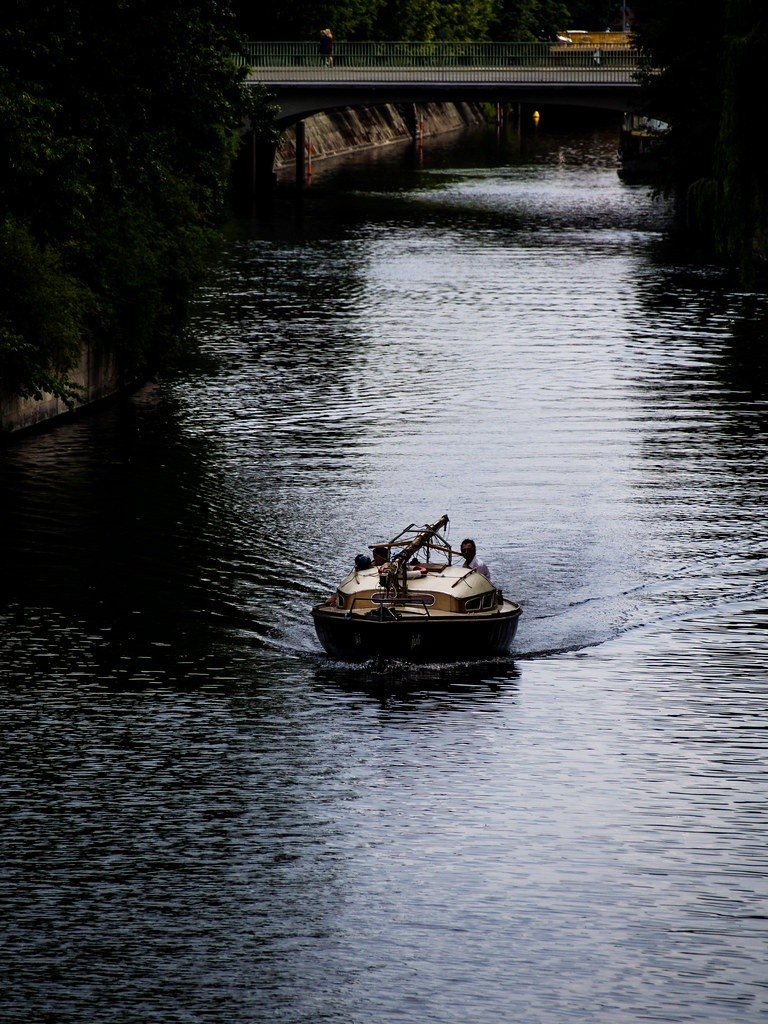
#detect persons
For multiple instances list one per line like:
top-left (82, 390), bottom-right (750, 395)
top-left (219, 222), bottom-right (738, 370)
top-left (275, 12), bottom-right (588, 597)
top-left (355, 554), bottom-right (372, 570)
top-left (461, 539), bottom-right (490, 580)
top-left (372, 547), bottom-right (391, 566)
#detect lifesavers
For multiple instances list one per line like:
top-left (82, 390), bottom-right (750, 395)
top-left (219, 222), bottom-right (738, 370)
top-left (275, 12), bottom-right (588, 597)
top-left (378, 562), bottom-right (427, 580)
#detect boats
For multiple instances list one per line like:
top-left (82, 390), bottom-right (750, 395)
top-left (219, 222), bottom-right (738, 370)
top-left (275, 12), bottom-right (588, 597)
top-left (306, 513), bottom-right (524, 662)
top-left (617, 110), bottom-right (669, 169)
